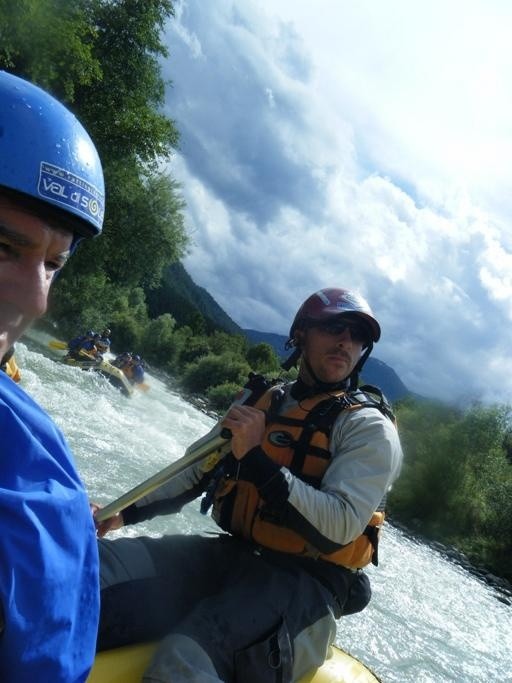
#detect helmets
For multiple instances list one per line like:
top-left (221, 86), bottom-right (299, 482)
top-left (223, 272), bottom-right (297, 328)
top-left (123, 352), bottom-right (144, 366)
top-left (0, 70), bottom-right (106, 256)
top-left (86, 329), bottom-right (111, 340)
top-left (290, 288), bottom-right (380, 351)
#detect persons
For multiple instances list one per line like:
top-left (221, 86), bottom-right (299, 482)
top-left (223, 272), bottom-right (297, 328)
top-left (1, 64), bottom-right (114, 683)
top-left (89, 288), bottom-right (402, 683)
top-left (61, 325), bottom-right (145, 388)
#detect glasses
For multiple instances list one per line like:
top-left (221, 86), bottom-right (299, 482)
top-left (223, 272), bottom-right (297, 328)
top-left (318, 317), bottom-right (370, 343)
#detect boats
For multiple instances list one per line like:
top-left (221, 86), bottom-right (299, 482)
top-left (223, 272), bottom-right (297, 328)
top-left (64, 352), bottom-right (133, 396)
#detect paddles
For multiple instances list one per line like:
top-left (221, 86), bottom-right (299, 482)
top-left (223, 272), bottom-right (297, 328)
top-left (137, 382), bottom-right (150, 392)
top-left (79, 348), bottom-right (97, 361)
top-left (49, 342), bottom-right (73, 350)
